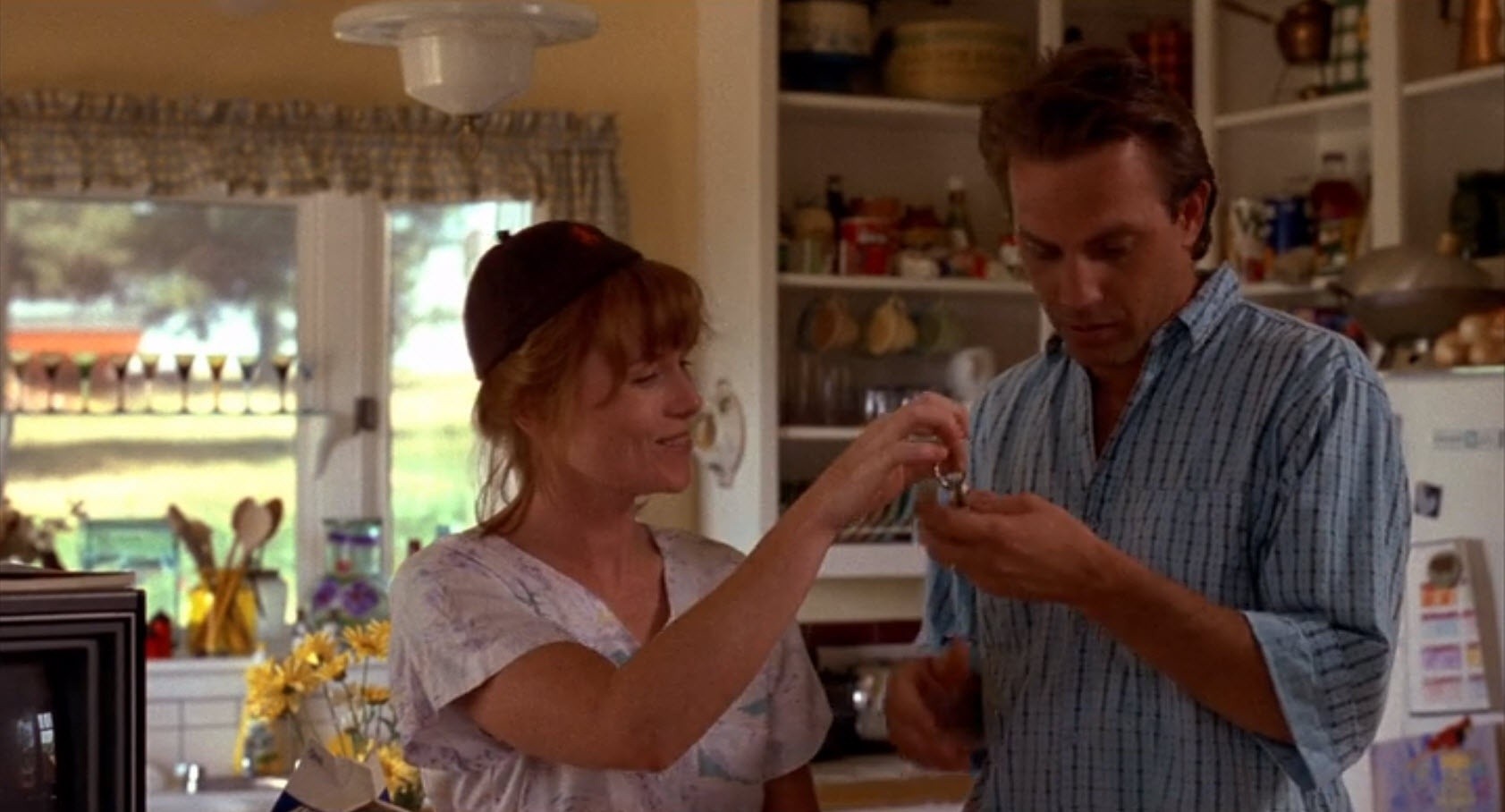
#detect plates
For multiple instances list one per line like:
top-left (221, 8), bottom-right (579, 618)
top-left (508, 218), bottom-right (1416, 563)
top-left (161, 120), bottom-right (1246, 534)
top-left (781, 463), bottom-right (922, 543)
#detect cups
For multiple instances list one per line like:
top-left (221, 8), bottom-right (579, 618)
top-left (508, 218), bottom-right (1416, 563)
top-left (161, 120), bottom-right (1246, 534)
top-left (783, 291), bottom-right (998, 427)
top-left (1229, 197), bottom-right (1268, 282)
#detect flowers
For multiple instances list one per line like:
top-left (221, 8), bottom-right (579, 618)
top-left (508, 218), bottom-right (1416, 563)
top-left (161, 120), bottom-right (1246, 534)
top-left (238, 615), bottom-right (425, 802)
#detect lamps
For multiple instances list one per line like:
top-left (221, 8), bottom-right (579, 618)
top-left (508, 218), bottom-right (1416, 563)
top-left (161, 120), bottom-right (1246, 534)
top-left (332, 1), bottom-right (600, 118)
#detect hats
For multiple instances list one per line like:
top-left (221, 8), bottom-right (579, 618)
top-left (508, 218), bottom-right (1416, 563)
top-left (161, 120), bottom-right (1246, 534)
top-left (463, 220), bottom-right (641, 377)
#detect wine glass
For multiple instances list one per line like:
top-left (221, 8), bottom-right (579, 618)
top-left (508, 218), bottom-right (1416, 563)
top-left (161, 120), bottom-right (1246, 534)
top-left (5, 351), bottom-right (35, 415)
top-left (107, 353), bottom-right (197, 415)
top-left (268, 355), bottom-right (294, 414)
top-left (237, 355), bottom-right (261, 416)
top-left (205, 354), bottom-right (227, 415)
top-left (39, 351), bottom-right (101, 415)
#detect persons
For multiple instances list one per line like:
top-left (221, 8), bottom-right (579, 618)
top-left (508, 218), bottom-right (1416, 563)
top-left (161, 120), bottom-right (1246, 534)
top-left (388, 219), bottom-right (968, 812)
top-left (886, 46), bottom-right (1413, 812)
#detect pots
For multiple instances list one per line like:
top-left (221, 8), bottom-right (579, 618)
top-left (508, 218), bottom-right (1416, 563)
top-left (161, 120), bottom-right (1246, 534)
top-left (1319, 231), bottom-right (1505, 346)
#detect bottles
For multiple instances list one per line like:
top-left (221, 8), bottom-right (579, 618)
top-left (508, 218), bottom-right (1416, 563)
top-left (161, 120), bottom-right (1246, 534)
top-left (781, 173), bottom-right (1030, 283)
top-left (119, 551), bottom-right (286, 659)
top-left (1263, 150), bottom-right (1364, 279)
top-left (304, 516), bottom-right (389, 632)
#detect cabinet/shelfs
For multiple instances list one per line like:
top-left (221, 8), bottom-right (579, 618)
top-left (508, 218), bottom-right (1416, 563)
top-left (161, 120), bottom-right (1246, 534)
top-left (694, 1), bottom-right (1505, 582)
